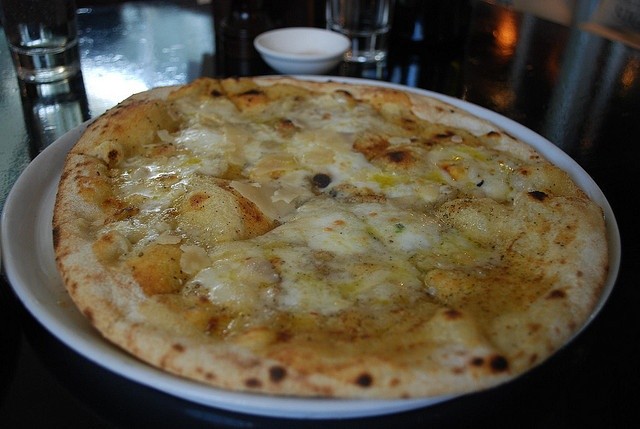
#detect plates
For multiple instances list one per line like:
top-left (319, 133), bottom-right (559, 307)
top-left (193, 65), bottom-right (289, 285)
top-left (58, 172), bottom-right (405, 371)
top-left (2, 74), bottom-right (622, 419)
top-left (253, 27), bottom-right (351, 74)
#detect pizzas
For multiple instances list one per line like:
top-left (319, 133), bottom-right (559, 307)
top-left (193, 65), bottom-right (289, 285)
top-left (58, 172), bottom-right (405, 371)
top-left (50, 73), bottom-right (608, 400)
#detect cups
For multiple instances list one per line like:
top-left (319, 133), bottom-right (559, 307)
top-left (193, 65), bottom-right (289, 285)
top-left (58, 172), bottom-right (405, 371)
top-left (0, 1), bottom-right (82, 85)
top-left (325, 0), bottom-right (389, 64)
